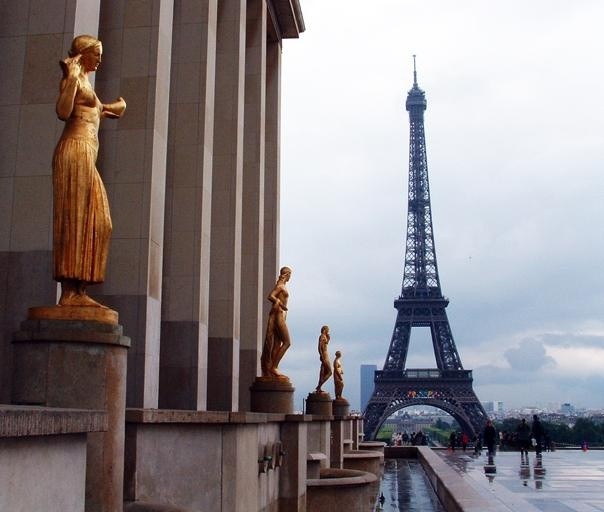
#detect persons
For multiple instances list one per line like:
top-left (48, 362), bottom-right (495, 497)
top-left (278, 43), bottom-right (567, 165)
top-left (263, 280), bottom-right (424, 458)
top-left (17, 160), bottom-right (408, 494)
top-left (52, 34), bottom-right (128, 307)
top-left (392, 429), bottom-right (428, 446)
top-left (446, 414), bottom-right (554, 456)
top-left (333, 350), bottom-right (345, 400)
top-left (580, 440), bottom-right (589, 452)
top-left (533, 453), bottom-right (546, 490)
top-left (484, 455), bottom-right (496, 484)
top-left (519, 449), bottom-right (531, 486)
top-left (315, 326), bottom-right (332, 392)
top-left (261, 266), bottom-right (291, 377)
